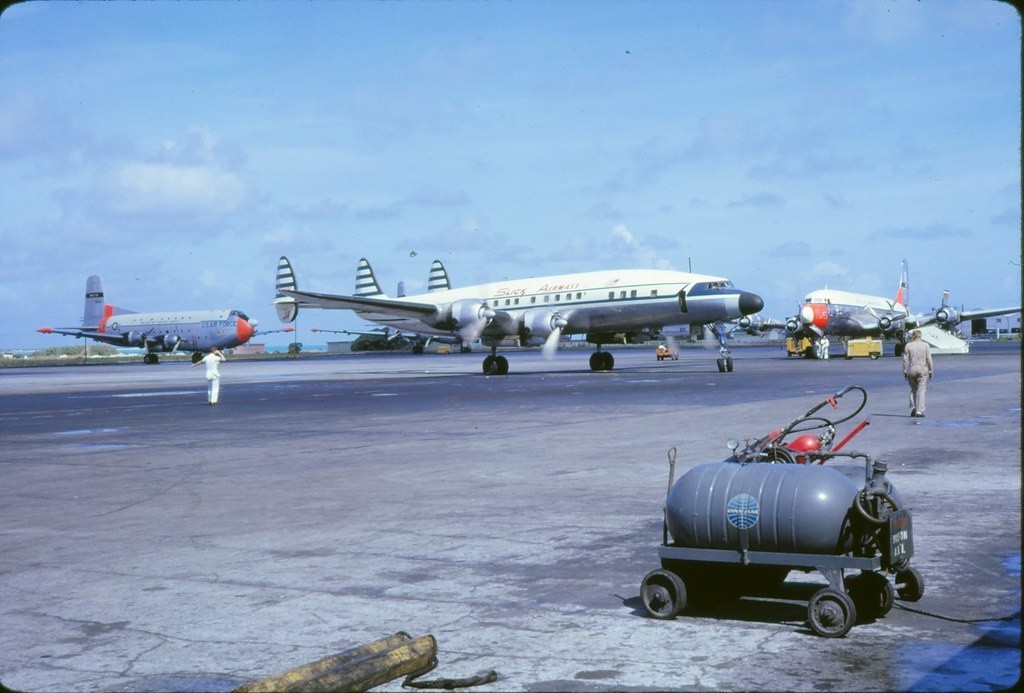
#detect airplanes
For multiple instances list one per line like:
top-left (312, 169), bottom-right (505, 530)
top-left (35, 275), bottom-right (258, 364)
top-left (728, 259), bottom-right (1021, 359)
top-left (272, 257), bottom-right (764, 374)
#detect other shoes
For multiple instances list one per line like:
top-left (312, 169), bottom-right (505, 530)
top-left (911, 408), bottom-right (925, 417)
top-left (209, 403), bottom-right (217, 406)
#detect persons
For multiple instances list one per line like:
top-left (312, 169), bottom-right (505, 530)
top-left (657, 343), bottom-right (666, 361)
top-left (820, 336), bottom-right (829, 360)
top-left (903, 330), bottom-right (934, 417)
top-left (812, 339), bottom-right (822, 358)
top-left (192, 347), bottom-right (226, 405)
